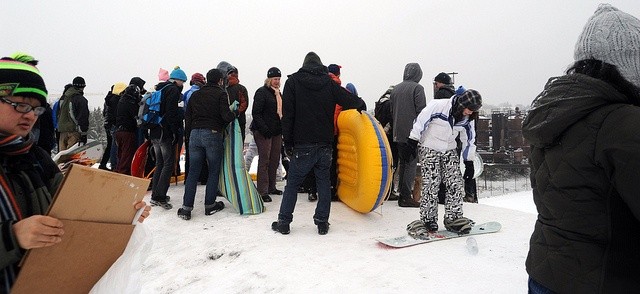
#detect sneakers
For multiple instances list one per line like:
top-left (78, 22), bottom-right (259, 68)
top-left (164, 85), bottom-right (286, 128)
top-left (178, 208), bottom-right (191, 220)
top-left (261, 193), bottom-right (272, 202)
top-left (314, 216), bottom-right (329, 234)
top-left (150, 196), bottom-right (170, 207)
top-left (444, 219), bottom-right (471, 233)
top-left (398, 197), bottom-right (419, 207)
top-left (308, 188), bottom-right (317, 200)
top-left (269, 189), bottom-right (283, 195)
top-left (424, 218), bottom-right (438, 231)
top-left (205, 200), bottom-right (225, 215)
top-left (272, 220), bottom-right (290, 234)
top-left (150, 196), bottom-right (172, 209)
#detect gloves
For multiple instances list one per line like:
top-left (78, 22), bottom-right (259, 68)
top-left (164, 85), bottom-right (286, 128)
top-left (78, 134), bottom-right (87, 147)
top-left (284, 146), bottom-right (294, 160)
top-left (463, 161), bottom-right (474, 183)
top-left (402, 138), bottom-right (418, 163)
top-left (356, 101), bottom-right (367, 114)
top-left (263, 130), bottom-right (272, 140)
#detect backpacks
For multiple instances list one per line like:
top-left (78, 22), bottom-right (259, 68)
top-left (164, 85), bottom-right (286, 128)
top-left (136, 83), bottom-right (181, 130)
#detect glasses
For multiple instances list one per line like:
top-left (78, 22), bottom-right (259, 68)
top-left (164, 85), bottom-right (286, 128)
top-left (0, 97), bottom-right (47, 116)
top-left (435, 83), bottom-right (437, 86)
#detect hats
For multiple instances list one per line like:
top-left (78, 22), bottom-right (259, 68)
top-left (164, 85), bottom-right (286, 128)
top-left (159, 68), bottom-right (169, 80)
top-left (0, 52), bottom-right (48, 107)
top-left (63, 84), bottom-right (72, 94)
top-left (434, 73), bottom-right (452, 84)
top-left (192, 73), bottom-right (204, 84)
top-left (268, 67), bottom-right (281, 78)
top-left (577, 3), bottom-right (640, 86)
top-left (458, 90), bottom-right (482, 110)
top-left (328, 64), bottom-right (340, 76)
top-left (169, 64), bottom-right (187, 82)
top-left (73, 77), bottom-right (86, 87)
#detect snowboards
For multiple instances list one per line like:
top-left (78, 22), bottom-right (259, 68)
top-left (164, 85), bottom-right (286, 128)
top-left (375, 221), bottom-right (501, 247)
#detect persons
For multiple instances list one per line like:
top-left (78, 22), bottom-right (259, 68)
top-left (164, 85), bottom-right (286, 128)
top-left (53, 84), bottom-right (73, 154)
top-left (97, 75), bottom-right (145, 176)
top-left (403, 89), bottom-right (482, 233)
top-left (271, 52), bottom-right (367, 235)
top-left (140, 67), bottom-right (186, 210)
top-left (309, 61), bottom-right (342, 203)
top-left (251, 67), bottom-right (283, 204)
top-left (520, 2), bottom-right (640, 294)
top-left (183, 72), bottom-right (206, 187)
top-left (376, 85), bottom-right (401, 201)
top-left (434, 73), bottom-right (478, 204)
top-left (392, 63), bottom-right (427, 207)
top-left (177, 69), bottom-right (226, 218)
top-left (58, 77), bottom-right (89, 153)
top-left (0, 51), bottom-right (151, 294)
top-left (218, 61), bottom-right (248, 152)
